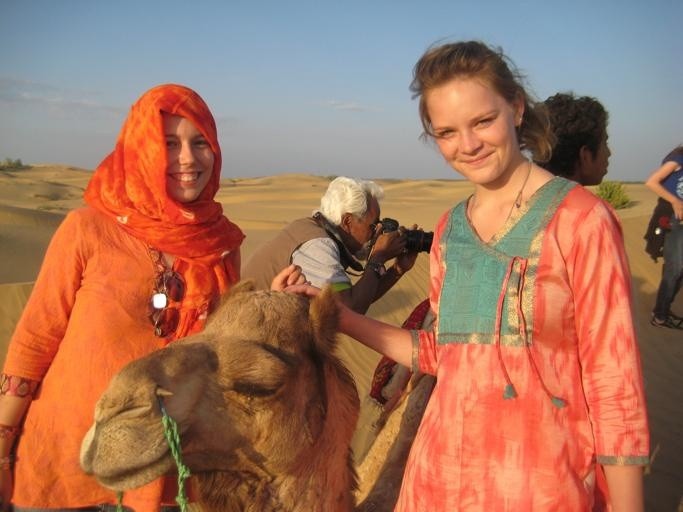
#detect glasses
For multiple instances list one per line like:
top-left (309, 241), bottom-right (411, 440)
top-left (148, 272), bottom-right (186, 337)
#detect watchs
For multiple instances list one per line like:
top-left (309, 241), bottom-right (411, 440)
top-left (363, 258), bottom-right (388, 279)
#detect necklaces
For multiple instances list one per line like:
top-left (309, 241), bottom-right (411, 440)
top-left (469, 161), bottom-right (532, 246)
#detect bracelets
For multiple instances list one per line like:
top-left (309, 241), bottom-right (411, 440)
top-left (1, 424), bottom-right (22, 440)
top-left (0, 455), bottom-right (13, 468)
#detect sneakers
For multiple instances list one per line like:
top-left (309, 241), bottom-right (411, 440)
top-left (652, 306), bottom-right (683, 329)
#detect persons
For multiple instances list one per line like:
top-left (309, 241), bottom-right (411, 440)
top-left (1, 81), bottom-right (314, 512)
top-left (279, 38), bottom-right (656, 512)
top-left (642, 138), bottom-right (683, 333)
top-left (239, 175), bottom-right (426, 316)
top-left (525, 88), bottom-right (614, 187)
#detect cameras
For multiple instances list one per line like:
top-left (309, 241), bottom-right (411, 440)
top-left (371, 217), bottom-right (434, 253)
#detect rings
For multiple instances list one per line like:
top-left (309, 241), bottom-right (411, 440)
top-left (400, 247), bottom-right (408, 257)
top-left (396, 228), bottom-right (404, 238)
